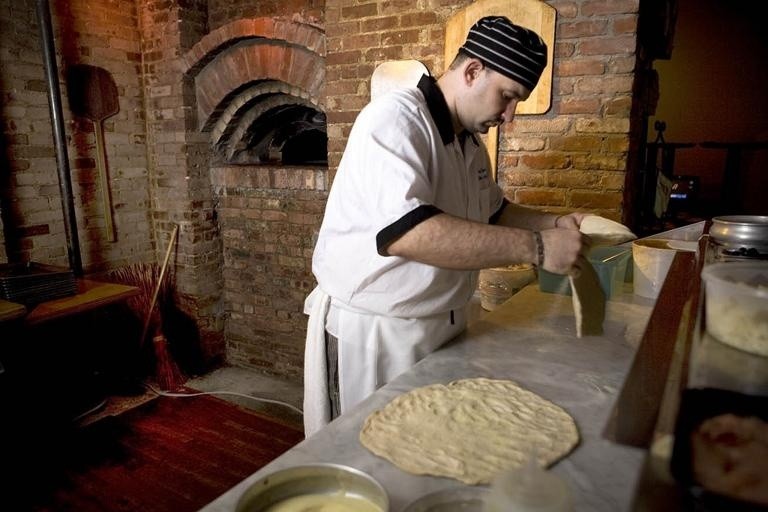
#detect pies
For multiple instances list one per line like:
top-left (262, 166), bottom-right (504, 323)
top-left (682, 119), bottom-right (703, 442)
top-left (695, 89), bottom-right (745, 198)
top-left (691, 413), bottom-right (768, 505)
top-left (567, 215), bottom-right (638, 339)
top-left (360, 377), bottom-right (579, 487)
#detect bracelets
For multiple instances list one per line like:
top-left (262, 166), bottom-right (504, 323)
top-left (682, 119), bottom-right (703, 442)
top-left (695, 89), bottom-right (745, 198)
top-left (534, 232), bottom-right (544, 269)
top-left (554, 211), bottom-right (576, 228)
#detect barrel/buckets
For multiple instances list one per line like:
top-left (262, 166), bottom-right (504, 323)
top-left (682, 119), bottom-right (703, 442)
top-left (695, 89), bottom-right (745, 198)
top-left (478, 267), bottom-right (535, 311)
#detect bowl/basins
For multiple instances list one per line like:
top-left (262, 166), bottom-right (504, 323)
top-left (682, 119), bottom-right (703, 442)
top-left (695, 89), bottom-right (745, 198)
top-left (232, 462), bottom-right (391, 512)
top-left (703, 213), bottom-right (768, 253)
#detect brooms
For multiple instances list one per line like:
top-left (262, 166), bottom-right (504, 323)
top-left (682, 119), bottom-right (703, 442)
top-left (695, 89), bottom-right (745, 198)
top-left (104, 264), bottom-right (189, 393)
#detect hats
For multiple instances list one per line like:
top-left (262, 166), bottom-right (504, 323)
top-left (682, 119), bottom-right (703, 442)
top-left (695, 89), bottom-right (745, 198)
top-left (457, 13), bottom-right (550, 92)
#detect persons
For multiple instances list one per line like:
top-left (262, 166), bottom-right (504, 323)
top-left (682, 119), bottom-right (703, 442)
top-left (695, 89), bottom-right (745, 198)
top-left (302, 16), bottom-right (594, 440)
